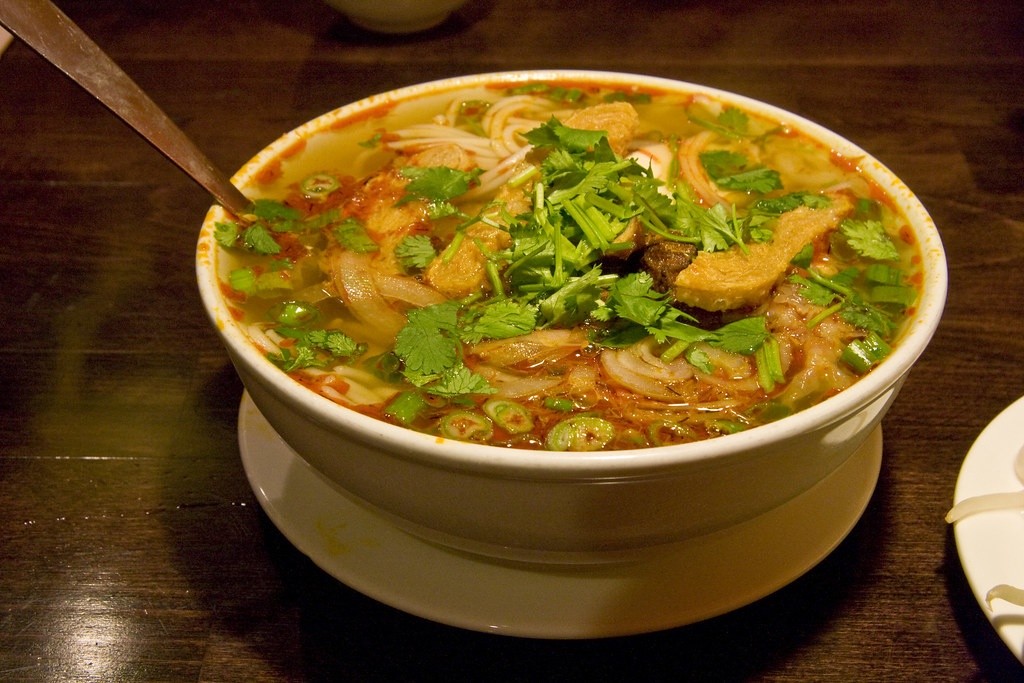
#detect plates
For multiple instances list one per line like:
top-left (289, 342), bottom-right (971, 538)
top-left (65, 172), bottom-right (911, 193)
top-left (952, 396), bottom-right (1024, 663)
top-left (240, 389), bottom-right (884, 638)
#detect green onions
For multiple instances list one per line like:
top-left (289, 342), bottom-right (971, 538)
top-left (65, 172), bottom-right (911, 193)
top-left (227, 171), bottom-right (916, 451)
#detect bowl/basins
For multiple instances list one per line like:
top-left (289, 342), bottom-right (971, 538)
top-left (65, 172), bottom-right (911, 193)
top-left (195, 70), bottom-right (949, 551)
top-left (329, 0), bottom-right (464, 34)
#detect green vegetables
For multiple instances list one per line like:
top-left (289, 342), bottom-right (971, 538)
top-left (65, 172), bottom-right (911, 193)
top-left (215, 86), bottom-right (900, 399)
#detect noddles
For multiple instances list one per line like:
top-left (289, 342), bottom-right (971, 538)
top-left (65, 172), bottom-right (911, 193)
top-left (214, 81), bottom-right (924, 451)
